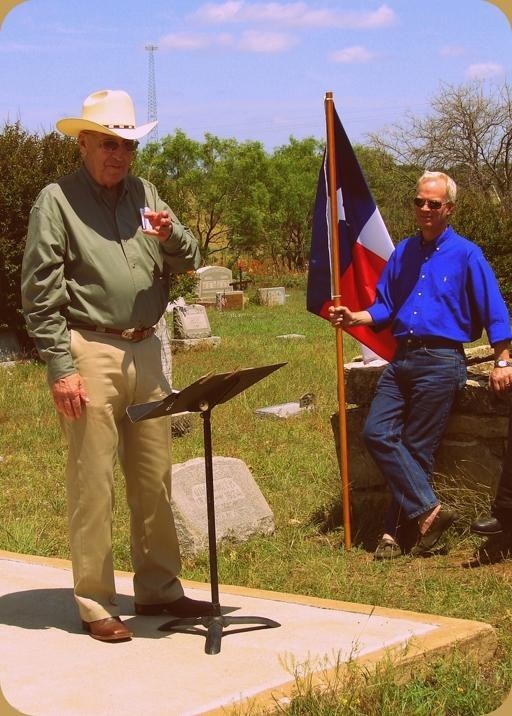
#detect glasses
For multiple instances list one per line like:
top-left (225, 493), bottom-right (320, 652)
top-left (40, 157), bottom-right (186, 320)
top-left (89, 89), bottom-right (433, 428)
top-left (410, 197), bottom-right (451, 209)
top-left (82, 131), bottom-right (141, 151)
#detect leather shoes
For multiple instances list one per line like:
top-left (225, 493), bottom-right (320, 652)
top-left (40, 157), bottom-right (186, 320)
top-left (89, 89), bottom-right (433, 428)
top-left (407, 502), bottom-right (458, 557)
top-left (135, 595), bottom-right (216, 616)
top-left (470, 515), bottom-right (509, 533)
top-left (374, 536), bottom-right (404, 561)
top-left (82, 614), bottom-right (134, 640)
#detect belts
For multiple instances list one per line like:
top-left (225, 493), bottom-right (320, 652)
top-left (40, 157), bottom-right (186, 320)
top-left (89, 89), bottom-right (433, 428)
top-left (398, 336), bottom-right (457, 349)
top-left (69, 314), bottom-right (165, 343)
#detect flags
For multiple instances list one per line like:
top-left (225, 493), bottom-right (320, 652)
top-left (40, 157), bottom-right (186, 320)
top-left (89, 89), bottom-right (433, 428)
top-left (307, 101), bottom-right (402, 365)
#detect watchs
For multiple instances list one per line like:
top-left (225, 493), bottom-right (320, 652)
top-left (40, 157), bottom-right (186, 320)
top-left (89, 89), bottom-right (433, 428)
top-left (493, 359), bottom-right (511, 368)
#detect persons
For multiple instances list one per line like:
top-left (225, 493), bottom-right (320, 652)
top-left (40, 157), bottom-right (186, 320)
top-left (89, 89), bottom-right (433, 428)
top-left (23, 91), bottom-right (219, 644)
top-left (471, 427), bottom-right (512, 537)
top-left (328, 170), bottom-right (512, 559)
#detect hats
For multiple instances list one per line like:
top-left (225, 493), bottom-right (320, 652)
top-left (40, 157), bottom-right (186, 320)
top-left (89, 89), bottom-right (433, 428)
top-left (57, 91), bottom-right (158, 138)
